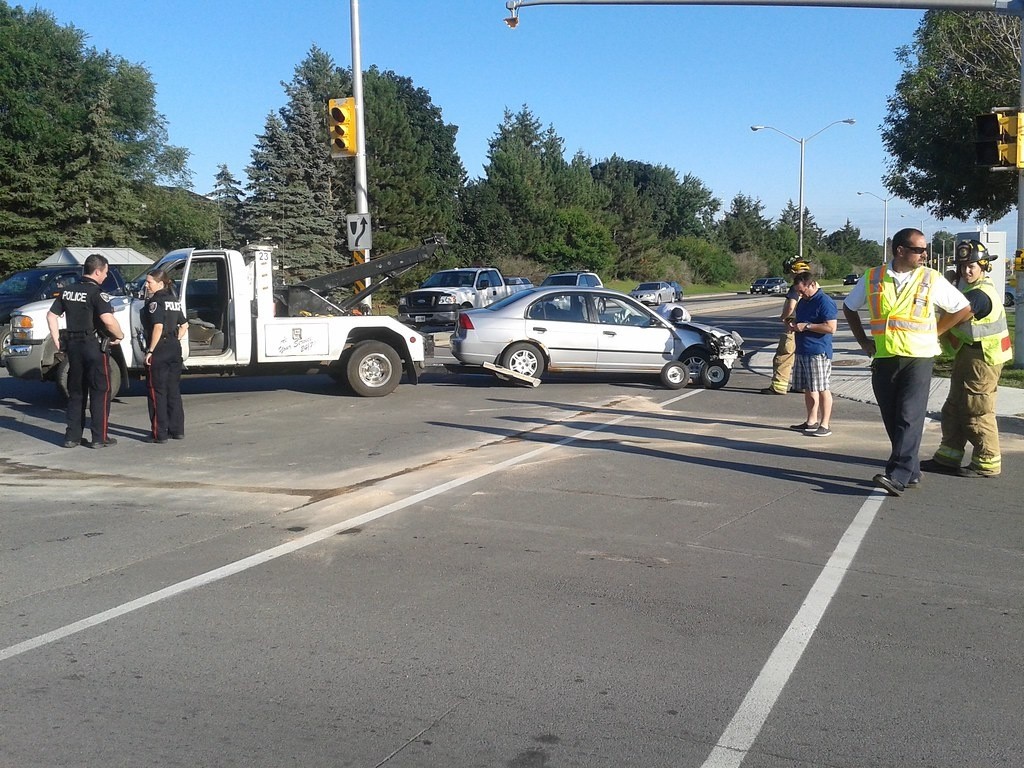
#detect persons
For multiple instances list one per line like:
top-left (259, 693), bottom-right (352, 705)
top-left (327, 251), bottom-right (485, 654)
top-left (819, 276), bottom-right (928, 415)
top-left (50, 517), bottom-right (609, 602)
top-left (46, 253), bottom-right (124, 449)
top-left (920, 239), bottom-right (1013, 478)
top-left (759, 254), bottom-right (837, 437)
top-left (656, 303), bottom-right (691, 323)
top-left (843, 228), bottom-right (970, 497)
top-left (140, 269), bottom-right (189, 444)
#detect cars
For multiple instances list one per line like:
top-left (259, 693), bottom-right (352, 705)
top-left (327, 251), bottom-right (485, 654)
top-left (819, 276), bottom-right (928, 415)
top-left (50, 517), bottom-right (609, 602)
top-left (749, 277), bottom-right (788, 295)
top-left (1003, 279), bottom-right (1016, 308)
top-left (842, 273), bottom-right (860, 286)
top-left (537, 269), bottom-right (607, 316)
top-left (626, 281), bottom-right (676, 306)
top-left (665, 281), bottom-right (684, 302)
top-left (449, 282), bottom-right (746, 391)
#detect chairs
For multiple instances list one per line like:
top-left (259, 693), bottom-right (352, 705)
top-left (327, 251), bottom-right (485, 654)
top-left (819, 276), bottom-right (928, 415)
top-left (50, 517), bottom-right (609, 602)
top-left (572, 301), bottom-right (586, 322)
top-left (189, 290), bottom-right (227, 356)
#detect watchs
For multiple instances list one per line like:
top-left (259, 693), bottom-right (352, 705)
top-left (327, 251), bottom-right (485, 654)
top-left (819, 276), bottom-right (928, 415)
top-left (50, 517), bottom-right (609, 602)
top-left (807, 323), bottom-right (811, 330)
top-left (146, 349), bottom-right (153, 354)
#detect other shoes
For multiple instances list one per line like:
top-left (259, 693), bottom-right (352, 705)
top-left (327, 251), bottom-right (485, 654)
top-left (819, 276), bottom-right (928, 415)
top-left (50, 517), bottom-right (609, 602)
top-left (958, 463), bottom-right (1000, 478)
top-left (761, 386), bottom-right (783, 395)
top-left (789, 386), bottom-right (805, 393)
top-left (919, 458), bottom-right (961, 475)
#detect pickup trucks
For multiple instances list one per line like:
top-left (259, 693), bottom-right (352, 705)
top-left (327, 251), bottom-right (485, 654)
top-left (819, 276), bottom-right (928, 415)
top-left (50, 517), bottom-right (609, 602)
top-left (0, 263), bottom-right (221, 373)
top-left (2, 238), bottom-right (456, 398)
top-left (397, 266), bottom-right (536, 344)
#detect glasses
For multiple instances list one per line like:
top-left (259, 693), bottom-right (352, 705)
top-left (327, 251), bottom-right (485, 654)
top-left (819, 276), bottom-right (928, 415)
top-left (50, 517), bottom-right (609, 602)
top-left (902, 245), bottom-right (928, 254)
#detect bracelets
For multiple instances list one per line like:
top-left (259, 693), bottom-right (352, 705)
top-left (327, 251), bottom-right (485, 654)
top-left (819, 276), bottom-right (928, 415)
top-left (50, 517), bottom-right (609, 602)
top-left (114, 334), bottom-right (124, 340)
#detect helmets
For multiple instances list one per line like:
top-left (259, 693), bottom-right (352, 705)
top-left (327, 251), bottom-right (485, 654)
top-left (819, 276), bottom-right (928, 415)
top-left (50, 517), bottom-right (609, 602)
top-left (950, 238), bottom-right (997, 266)
top-left (782, 253), bottom-right (812, 274)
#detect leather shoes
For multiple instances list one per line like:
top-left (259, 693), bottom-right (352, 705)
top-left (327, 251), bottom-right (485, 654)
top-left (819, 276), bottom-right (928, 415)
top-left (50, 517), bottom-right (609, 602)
top-left (65, 438), bottom-right (88, 448)
top-left (91, 438), bottom-right (117, 448)
top-left (872, 473), bottom-right (905, 497)
top-left (167, 430), bottom-right (185, 439)
top-left (140, 435), bottom-right (167, 444)
top-left (908, 478), bottom-right (921, 488)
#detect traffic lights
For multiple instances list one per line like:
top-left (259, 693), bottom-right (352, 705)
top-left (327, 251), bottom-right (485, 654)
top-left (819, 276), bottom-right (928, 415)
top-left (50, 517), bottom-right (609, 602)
top-left (997, 112), bottom-right (1024, 169)
top-left (328, 97), bottom-right (357, 158)
top-left (972, 111), bottom-right (1005, 167)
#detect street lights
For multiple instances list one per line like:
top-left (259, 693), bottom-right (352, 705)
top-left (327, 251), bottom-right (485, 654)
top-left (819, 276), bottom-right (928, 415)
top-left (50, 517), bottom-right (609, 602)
top-left (924, 226), bottom-right (956, 278)
top-left (856, 191), bottom-right (902, 265)
top-left (901, 214), bottom-right (937, 233)
top-left (750, 117), bottom-right (857, 260)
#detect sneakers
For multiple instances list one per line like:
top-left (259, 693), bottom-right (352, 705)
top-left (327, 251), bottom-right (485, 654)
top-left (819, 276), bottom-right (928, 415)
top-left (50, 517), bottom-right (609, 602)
top-left (812, 425), bottom-right (833, 437)
top-left (789, 422), bottom-right (819, 432)
